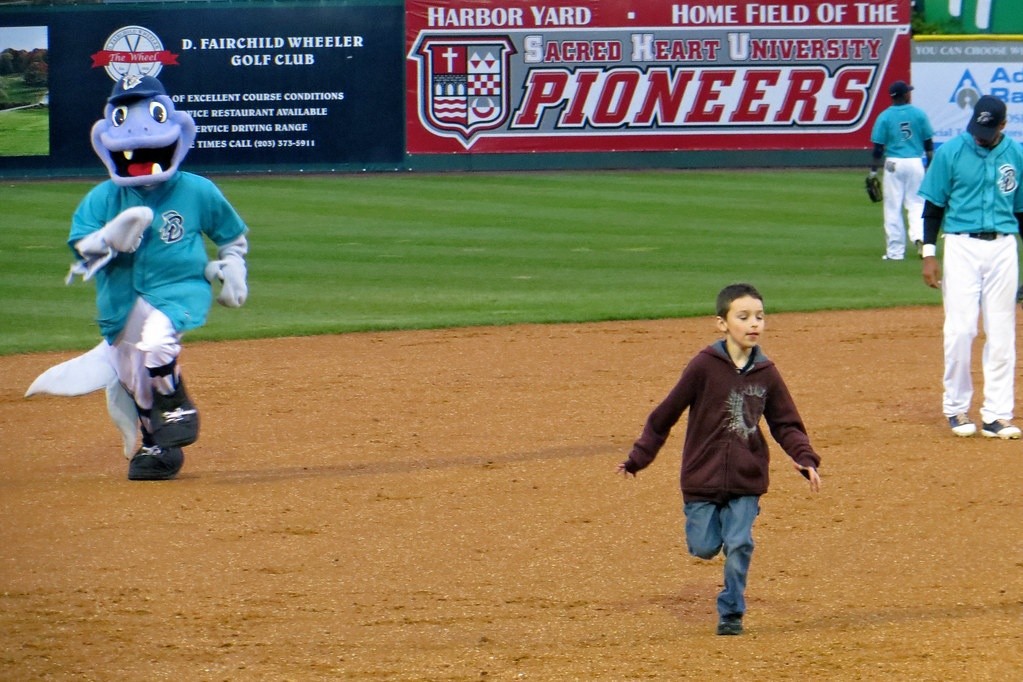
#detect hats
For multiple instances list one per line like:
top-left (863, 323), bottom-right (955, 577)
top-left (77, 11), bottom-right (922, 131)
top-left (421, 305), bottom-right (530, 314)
top-left (967, 94), bottom-right (1007, 142)
top-left (889, 81), bottom-right (913, 98)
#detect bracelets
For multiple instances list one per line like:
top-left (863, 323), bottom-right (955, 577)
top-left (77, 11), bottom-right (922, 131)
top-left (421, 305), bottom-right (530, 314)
top-left (922, 244), bottom-right (936, 257)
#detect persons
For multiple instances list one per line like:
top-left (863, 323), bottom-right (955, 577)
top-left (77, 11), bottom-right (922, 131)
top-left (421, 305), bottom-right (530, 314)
top-left (615, 283), bottom-right (823, 635)
top-left (867, 79), bottom-right (935, 261)
top-left (918, 95), bottom-right (1023, 441)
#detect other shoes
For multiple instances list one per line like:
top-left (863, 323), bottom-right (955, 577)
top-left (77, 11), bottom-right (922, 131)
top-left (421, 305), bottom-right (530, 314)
top-left (916, 239), bottom-right (923, 260)
top-left (882, 255), bottom-right (891, 260)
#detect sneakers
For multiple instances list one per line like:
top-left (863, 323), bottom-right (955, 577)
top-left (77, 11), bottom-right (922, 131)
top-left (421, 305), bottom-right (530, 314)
top-left (717, 615), bottom-right (743, 635)
top-left (950, 413), bottom-right (976, 437)
top-left (981, 419), bottom-right (1021, 439)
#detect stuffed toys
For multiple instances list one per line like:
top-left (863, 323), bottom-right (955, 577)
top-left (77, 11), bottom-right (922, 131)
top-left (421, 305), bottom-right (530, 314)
top-left (21, 76), bottom-right (251, 482)
